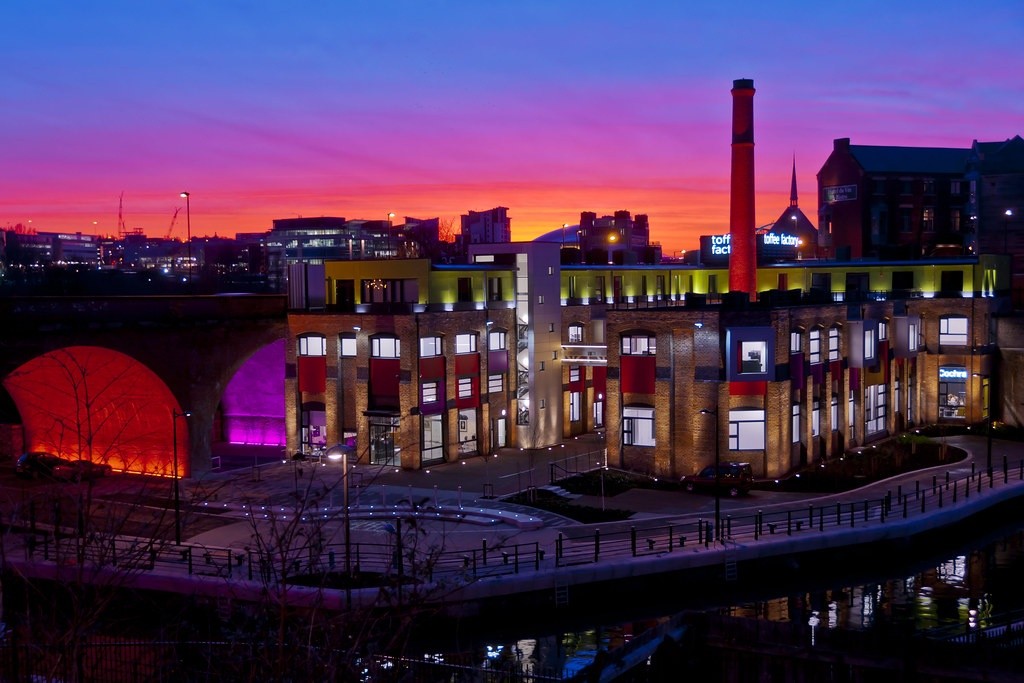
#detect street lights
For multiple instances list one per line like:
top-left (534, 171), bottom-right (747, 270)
top-left (172, 407), bottom-right (194, 546)
top-left (327, 443), bottom-right (352, 608)
top-left (674, 250), bottom-right (685, 258)
top-left (700, 405), bottom-right (720, 540)
top-left (562, 223), bottom-right (568, 243)
top-left (387, 212), bottom-right (396, 259)
top-left (182, 192), bottom-right (191, 284)
top-left (973, 372), bottom-right (994, 477)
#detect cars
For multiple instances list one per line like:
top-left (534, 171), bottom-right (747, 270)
top-left (54, 458), bottom-right (113, 480)
top-left (17, 452), bottom-right (70, 478)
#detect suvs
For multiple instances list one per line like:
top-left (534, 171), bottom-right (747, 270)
top-left (680, 462), bottom-right (755, 498)
